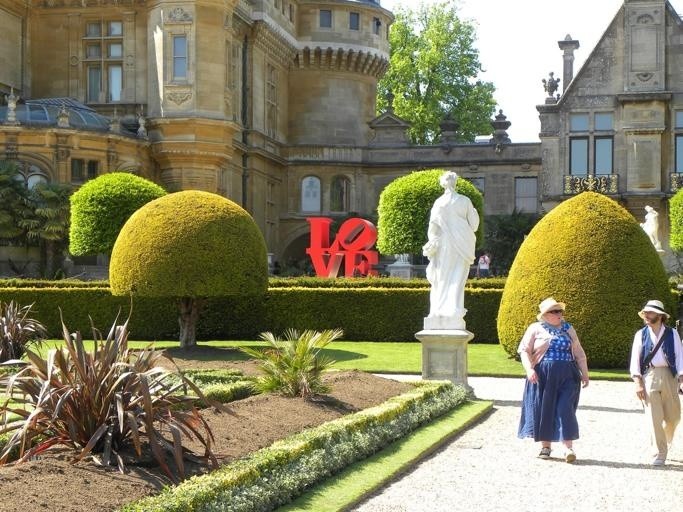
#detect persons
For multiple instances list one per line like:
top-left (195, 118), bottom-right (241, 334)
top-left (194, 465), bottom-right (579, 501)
top-left (420, 171), bottom-right (480, 330)
top-left (517, 297), bottom-right (589, 463)
top-left (630, 299), bottom-right (683, 468)
top-left (477, 253), bottom-right (492, 278)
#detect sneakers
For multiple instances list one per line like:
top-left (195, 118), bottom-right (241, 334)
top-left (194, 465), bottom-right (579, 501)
top-left (566, 448), bottom-right (576, 462)
top-left (653, 458), bottom-right (665, 466)
top-left (539, 447), bottom-right (552, 459)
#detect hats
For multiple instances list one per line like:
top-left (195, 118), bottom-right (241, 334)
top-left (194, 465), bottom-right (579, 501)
top-left (638, 300), bottom-right (671, 319)
top-left (537, 297), bottom-right (566, 320)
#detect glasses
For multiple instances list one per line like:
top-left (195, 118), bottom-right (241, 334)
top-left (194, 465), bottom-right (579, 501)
top-left (547, 309), bottom-right (564, 314)
